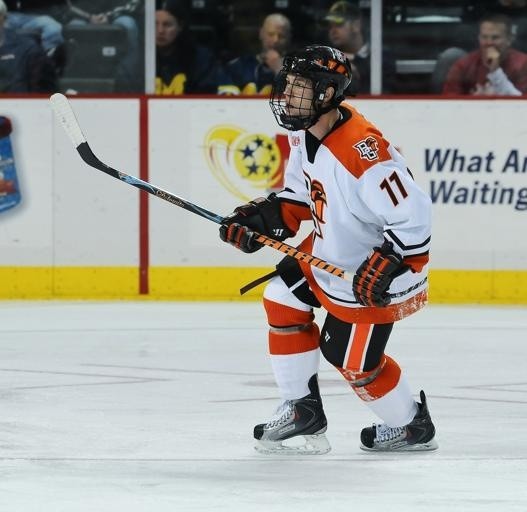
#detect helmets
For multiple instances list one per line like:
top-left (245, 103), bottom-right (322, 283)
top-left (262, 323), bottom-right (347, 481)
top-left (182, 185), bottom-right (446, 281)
top-left (283, 46), bottom-right (353, 109)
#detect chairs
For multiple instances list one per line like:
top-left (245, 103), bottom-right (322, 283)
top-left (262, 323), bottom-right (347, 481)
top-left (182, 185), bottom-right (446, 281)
top-left (57, 22), bottom-right (127, 92)
top-left (430, 46), bottom-right (467, 92)
top-left (185, 26), bottom-right (261, 77)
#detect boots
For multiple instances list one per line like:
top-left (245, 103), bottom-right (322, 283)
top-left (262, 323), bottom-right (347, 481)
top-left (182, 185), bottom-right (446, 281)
top-left (360, 390), bottom-right (436, 449)
top-left (253, 373), bottom-right (327, 441)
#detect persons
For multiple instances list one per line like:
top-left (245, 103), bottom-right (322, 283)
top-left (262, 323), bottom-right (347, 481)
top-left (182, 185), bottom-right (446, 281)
top-left (323, 0), bottom-right (401, 94)
top-left (156, 1), bottom-right (226, 94)
top-left (226, 13), bottom-right (293, 95)
top-left (441, 10), bottom-right (527, 96)
top-left (219, 45), bottom-right (436, 450)
top-left (0, 0), bottom-right (145, 94)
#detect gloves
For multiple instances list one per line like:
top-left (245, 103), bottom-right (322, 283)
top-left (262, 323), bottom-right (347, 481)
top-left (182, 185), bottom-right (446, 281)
top-left (351, 246), bottom-right (408, 308)
top-left (219, 192), bottom-right (294, 253)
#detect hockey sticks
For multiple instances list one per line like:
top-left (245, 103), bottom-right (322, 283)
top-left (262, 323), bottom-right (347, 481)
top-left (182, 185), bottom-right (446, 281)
top-left (50, 92), bottom-right (356, 287)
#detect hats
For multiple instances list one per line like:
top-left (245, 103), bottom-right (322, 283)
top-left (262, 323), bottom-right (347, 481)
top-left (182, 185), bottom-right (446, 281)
top-left (321, 0), bottom-right (361, 24)
